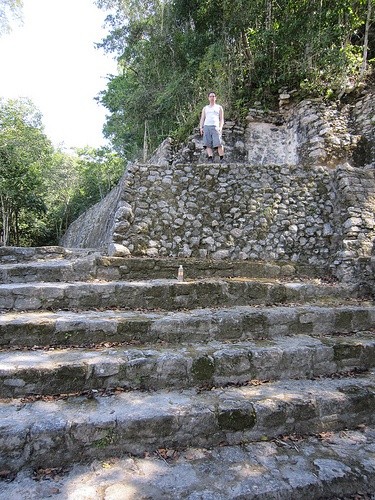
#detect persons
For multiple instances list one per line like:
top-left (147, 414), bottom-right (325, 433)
top-left (199, 92), bottom-right (229, 164)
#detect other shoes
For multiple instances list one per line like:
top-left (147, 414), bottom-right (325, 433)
top-left (208, 158), bottom-right (214, 164)
top-left (219, 159), bottom-right (224, 164)
